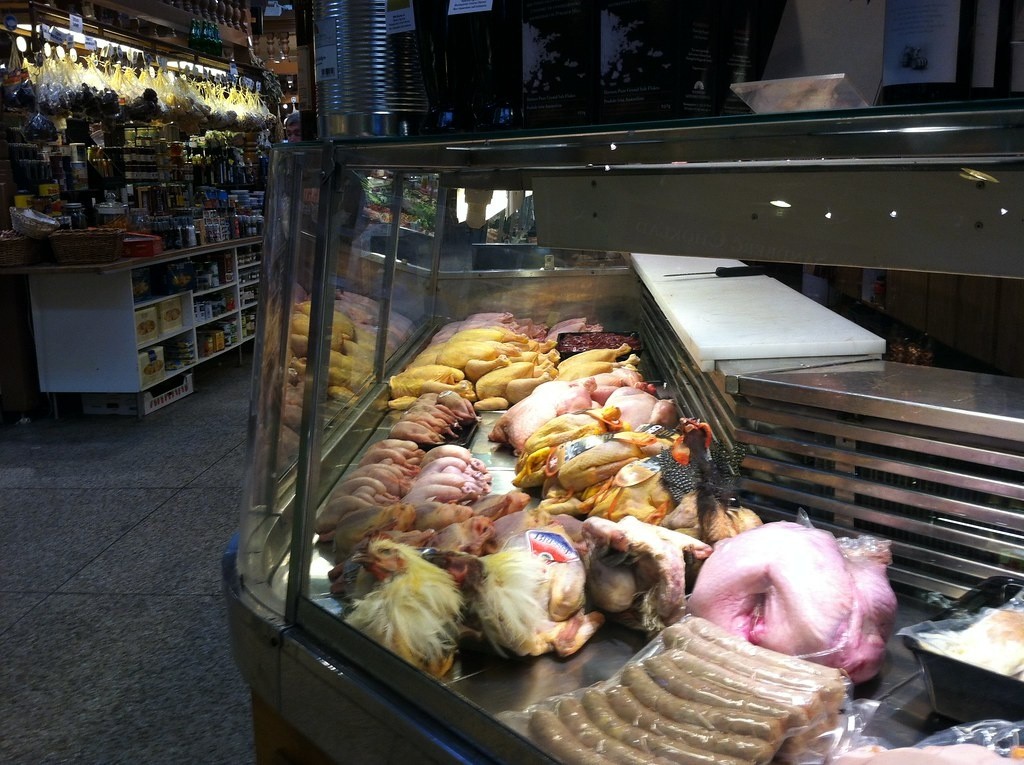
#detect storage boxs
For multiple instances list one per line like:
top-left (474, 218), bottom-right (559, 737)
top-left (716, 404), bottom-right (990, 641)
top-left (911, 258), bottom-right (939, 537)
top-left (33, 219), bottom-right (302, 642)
top-left (148, 260), bottom-right (195, 296)
top-left (154, 296), bottom-right (183, 334)
top-left (132, 267), bottom-right (151, 303)
top-left (139, 345), bottom-right (166, 383)
top-left (195, 329), bottom-right (225, 353)
top-left (79, 371), bottom-right (193, 416)
top-left (135, 305), bottom-right (158, 344)
top-left (123, 236), bottom-right (164, 257)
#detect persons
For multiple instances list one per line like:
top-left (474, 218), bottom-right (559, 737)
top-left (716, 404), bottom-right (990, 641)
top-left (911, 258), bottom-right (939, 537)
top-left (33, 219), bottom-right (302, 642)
top-left (286, 112), bottom-right (367, 245)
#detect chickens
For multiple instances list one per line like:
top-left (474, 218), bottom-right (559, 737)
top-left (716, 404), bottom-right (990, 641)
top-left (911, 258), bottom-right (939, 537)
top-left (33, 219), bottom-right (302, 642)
top-left (277, 300), bottom-right (393, 470)
top-left (308, 326), bottom-right (762, 677)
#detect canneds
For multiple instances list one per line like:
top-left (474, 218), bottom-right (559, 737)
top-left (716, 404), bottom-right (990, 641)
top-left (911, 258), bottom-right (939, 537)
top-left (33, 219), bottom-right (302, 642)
top-left (124, 127), bottom-right (160, 184)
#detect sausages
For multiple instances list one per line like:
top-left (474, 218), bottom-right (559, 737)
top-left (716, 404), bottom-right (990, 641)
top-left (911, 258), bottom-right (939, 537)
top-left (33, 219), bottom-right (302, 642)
top-left (529, 615), bottom-right (846, 765)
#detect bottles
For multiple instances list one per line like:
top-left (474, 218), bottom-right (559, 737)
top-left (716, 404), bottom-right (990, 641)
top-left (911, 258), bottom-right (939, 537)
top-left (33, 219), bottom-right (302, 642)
top-left (870, 273), bottom-right (885, 304)
top-left (150, 165), bottom-right (196, 252)
top-left (188, 17), bottom-right (225, 56)
top-left (126, 208), bottom-right (152, 235)
top-left (52, 201), bottom-right (90, 230)
top-left (86, 145), bottom-right (114, 177)
top-left (94, 193), bottom-right (129, 229)
top-left (230, 216), bottom-right (267, 239)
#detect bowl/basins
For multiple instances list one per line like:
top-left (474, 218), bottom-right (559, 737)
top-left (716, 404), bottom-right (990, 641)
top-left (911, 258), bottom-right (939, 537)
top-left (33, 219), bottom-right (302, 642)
top-left (231, 189), bottom-right (266, 215)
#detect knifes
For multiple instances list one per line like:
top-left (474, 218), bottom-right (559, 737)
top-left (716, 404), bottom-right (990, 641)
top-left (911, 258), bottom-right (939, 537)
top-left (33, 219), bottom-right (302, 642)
top-left (664, 266), bottom-right (764, 278)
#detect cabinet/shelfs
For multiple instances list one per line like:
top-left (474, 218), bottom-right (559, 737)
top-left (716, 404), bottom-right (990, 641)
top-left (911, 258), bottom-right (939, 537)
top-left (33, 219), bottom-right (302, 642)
top-left (0, 235), bottom-right (261, 424)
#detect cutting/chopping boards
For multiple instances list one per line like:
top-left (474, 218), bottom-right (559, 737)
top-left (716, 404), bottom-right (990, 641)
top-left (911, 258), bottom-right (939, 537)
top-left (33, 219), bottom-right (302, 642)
top-left (629, 252), bottom-right (886, 373)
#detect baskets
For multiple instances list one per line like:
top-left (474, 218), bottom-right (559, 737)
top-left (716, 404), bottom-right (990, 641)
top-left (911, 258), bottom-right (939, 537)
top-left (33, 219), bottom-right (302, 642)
top-left (0, 235), bottom-right (36, 266)
top-left (9, 206), bottom-right (61, 240)
top-left (48, 228), bottom-right (126, 263)
